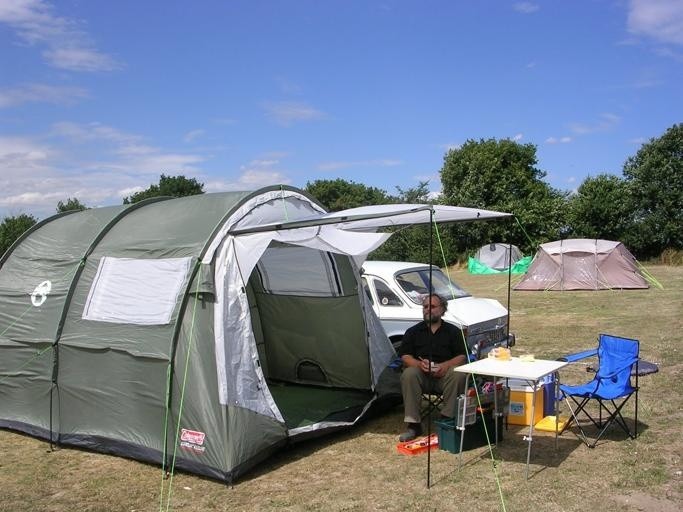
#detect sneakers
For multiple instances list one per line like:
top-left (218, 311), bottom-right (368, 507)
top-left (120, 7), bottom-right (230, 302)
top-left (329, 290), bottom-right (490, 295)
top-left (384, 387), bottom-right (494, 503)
top-left (399, 429), bottom-right (423, 442)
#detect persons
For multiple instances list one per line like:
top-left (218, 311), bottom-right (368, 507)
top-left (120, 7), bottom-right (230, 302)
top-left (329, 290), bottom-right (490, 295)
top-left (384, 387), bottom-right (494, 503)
top-left (397, 294), bottom-right (467, 442)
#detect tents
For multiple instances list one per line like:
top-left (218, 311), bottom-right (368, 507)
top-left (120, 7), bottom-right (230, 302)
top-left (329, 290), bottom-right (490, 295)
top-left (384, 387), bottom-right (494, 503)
top-left (474, 242), bottom-right (523, 270)
top-left (1, 184), bottom-right (516, 490)
top-left (513, 238), bottom-right (649, 289)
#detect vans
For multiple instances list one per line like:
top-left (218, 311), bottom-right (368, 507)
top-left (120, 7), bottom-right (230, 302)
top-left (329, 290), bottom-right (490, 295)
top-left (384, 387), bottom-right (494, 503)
top-left (357, 259), bottom-right (515, 359)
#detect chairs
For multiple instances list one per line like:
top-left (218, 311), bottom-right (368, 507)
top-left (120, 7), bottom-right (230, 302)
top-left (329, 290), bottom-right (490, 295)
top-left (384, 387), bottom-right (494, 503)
top-left (420, 388), bottom-right (444, 420)
top-left (558, 333), bottom-right (641, 447)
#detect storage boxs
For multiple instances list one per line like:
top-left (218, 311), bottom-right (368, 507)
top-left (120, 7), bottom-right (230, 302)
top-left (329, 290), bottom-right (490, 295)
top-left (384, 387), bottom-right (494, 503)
top-left (496, 373), bottom-right (557, 426)
top-left (433, 408), bottom-right (504, 454)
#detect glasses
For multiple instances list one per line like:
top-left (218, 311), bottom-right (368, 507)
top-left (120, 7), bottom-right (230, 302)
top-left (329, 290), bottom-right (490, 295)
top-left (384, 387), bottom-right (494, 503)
top-left (424, 305), bottom-right (442, 308)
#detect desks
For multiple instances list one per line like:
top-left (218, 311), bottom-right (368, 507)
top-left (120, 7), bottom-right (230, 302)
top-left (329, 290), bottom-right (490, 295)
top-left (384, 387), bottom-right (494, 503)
top-left (453, 356), bottom-right (570, 483)
